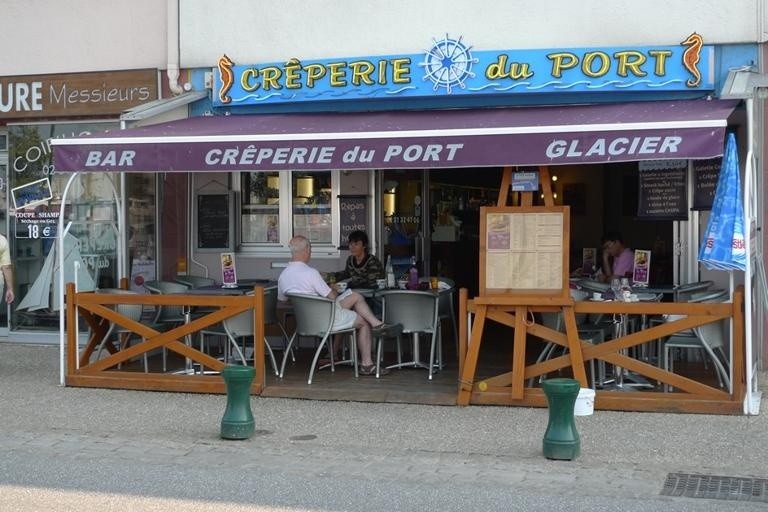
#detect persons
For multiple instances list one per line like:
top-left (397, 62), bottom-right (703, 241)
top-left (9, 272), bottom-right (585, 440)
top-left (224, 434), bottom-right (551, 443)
top-left (0, 234), bottom-right (16, 311)
top-left (275, 234), bottom-right (405, 377)
top-left (321, 229), bottom-right (387, 367)
top-left (599, 233), bottom-right (635, 282)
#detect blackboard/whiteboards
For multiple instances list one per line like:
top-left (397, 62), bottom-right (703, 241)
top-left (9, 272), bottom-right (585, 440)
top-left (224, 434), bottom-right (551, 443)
top-left (195, 190), bottom-right (236, 254)
top-left (633, 157), bottom-right (722, 221)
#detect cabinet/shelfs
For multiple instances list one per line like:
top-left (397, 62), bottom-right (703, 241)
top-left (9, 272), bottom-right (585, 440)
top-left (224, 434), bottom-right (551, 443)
top-left (1, 127), bottom-right (115, 330)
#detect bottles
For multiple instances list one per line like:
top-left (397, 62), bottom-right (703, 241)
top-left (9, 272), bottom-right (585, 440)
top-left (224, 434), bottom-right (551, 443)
top-left (408, 255), bottom-right (419, 290)
top-left (385, 255), bottom-right (395, 289)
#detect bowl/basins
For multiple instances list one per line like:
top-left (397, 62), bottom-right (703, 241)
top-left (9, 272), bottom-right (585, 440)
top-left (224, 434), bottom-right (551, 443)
top-left (592, 292), bottom-right (603, 299)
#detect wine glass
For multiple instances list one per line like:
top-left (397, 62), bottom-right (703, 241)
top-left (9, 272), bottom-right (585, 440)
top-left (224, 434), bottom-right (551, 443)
top-left (611, 278), bottom-right (629, 301)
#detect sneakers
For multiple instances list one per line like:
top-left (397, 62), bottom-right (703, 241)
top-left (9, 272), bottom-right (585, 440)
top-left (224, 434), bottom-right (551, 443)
top-left (371, 323), bottom-right (403, 337)
top-left (359, 365), bottom-right (390, 375)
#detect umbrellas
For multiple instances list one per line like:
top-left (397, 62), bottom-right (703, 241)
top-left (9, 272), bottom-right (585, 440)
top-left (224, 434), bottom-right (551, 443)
top-left (695, 132), bottom-right (747, 397)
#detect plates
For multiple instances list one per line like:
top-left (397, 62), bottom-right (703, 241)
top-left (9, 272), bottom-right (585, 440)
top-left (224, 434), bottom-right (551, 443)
top-left (636, 293), bottom-right (656, 301)
top-left (589, 298), bottom-right (605, 301)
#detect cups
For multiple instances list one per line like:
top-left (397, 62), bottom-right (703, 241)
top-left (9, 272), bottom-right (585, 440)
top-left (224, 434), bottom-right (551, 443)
top-left (376, 279), bottom-right (387, 288)
top-left (429, 276), bottom-right (439, 290)
top-left (624, 291), bottom-right (637, 303)
top-left (336, 283), bottom-right (347, 294)
top-left (398, 280), bottom-right (408, 290)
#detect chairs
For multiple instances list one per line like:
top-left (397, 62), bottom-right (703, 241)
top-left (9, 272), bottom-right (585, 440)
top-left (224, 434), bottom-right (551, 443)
top-left (420, 277), bottom-right (455, 324)
top-left (279, 292), bottom-right (359, 384)
top-left (223, 292), bottom-right (279, 377)
top-left (175, 276), bottom-right (223, 354)
top-left (143, 280), bottom-right (210, 355)
top-left (245, 282), bottom-right (298, 362)
top-left (282, 306), bottom-right (296, 356)
top-left (375, 290), bottom-right (442, 381)
top-left (528, 272), bottom-right (731, 393)
top-left (95, 288), bottom-right (166, 374)
